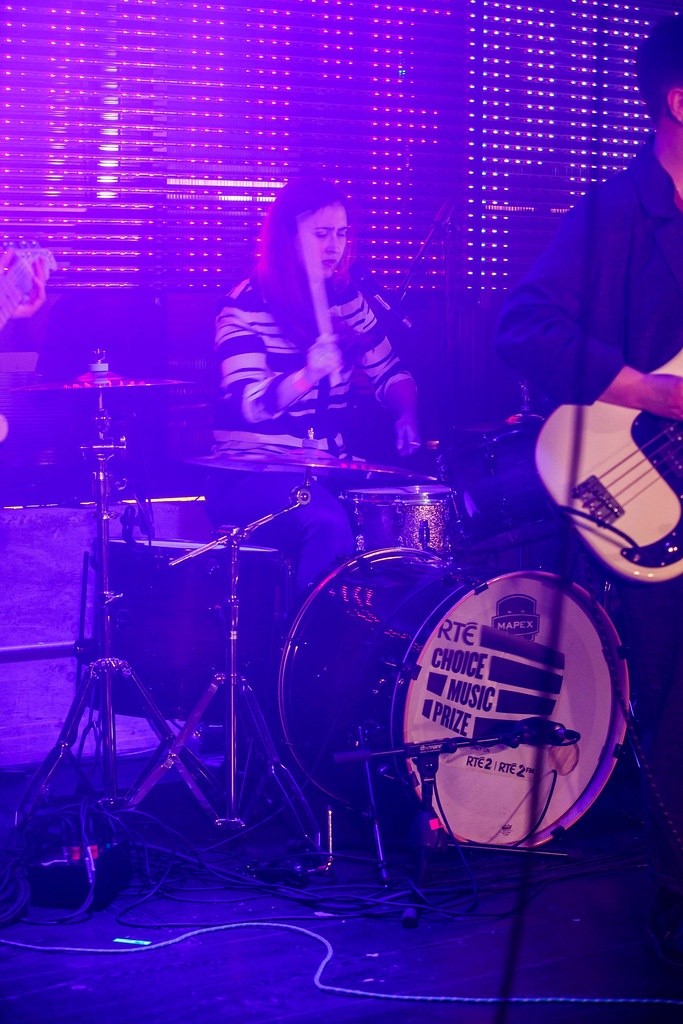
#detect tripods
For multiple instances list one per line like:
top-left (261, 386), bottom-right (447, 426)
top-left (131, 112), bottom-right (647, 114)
top-left (0, 389), bottom-right (547, 927)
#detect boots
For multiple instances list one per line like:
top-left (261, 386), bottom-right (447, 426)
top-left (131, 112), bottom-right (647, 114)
top-left (609, 683), bottom-right (666, 830)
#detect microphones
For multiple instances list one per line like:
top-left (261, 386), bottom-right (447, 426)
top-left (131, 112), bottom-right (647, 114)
top-left (374, 294), bottom-right (416, 332)
top-left (547, 724), bottom-right (580, 745)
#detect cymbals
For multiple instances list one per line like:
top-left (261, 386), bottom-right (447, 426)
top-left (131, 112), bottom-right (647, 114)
top-left (183, 440), bottom-right (440, 483)
top-left (7, 358), bottom-right (195, 397)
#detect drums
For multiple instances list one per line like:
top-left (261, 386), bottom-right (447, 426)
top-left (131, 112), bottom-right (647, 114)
top-left (338, 484), bottom-right (456, 563)
top-left (276, 539), bottom-right (634, 850)
top-left (78, 535), bottom-right (284, 725)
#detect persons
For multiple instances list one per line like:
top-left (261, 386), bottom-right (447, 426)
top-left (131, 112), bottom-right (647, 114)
top-left (210, 180), bottom-right (421, 596)
top-left (0, 246), bottom-right (49, 319)
top-left (497, 18), bottom-right (682, 969)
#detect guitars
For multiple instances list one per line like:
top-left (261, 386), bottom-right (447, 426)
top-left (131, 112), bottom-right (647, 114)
top-left (532, 344), bottom-right (683, 586)
